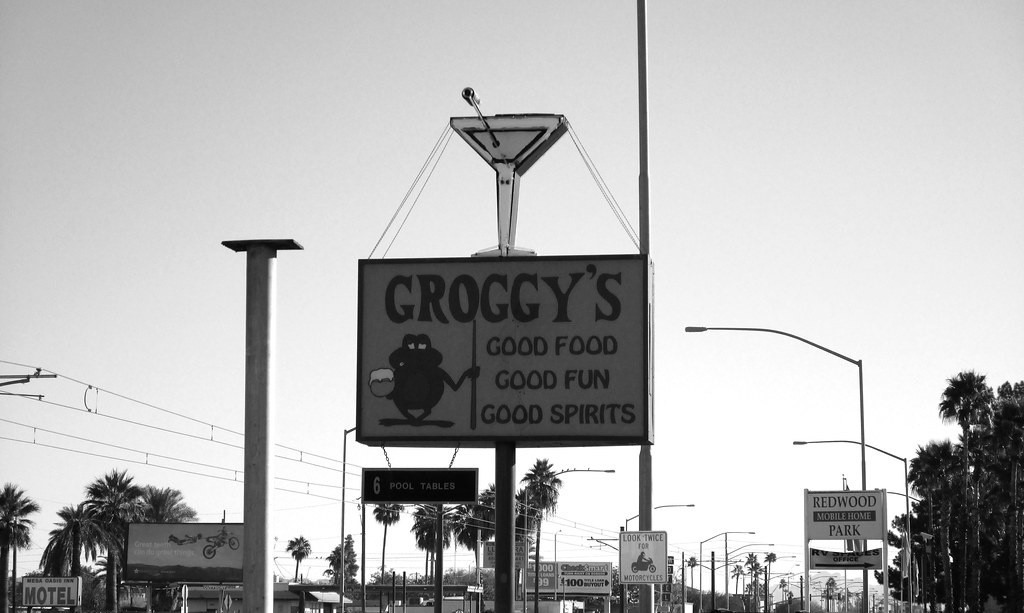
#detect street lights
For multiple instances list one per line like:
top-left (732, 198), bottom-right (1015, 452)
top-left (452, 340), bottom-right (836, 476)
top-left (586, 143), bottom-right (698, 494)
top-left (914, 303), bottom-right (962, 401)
top-left (684, 327), bottom-right (868, 612)
top-left (523, 468), bottom-right (615, 612)
top-left (700, 531), bottom-right (894, 612)
top-left (793, 440), bottom-right (913, 613)
top-left (625, 504), bottom-right (695, 613)
